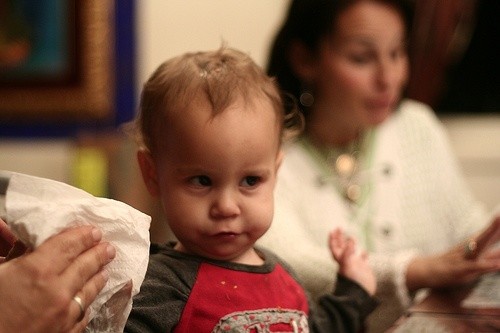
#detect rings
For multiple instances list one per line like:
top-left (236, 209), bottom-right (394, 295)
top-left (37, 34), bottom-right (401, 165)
top-left (73, 296), bottom-right (86, 314)
top-left (462, 240), bottom-right (480, 254)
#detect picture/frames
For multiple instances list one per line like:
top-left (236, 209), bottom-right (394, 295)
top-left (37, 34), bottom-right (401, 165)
top-left (0, 0), bottom-right (136, 142)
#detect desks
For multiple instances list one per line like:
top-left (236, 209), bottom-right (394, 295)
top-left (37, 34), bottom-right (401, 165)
top-left (384, 273), bottom-right (500, 333)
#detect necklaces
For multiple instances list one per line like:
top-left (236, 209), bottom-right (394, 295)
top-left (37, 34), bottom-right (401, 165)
top-left (300, 137), bottom-right (377, 201)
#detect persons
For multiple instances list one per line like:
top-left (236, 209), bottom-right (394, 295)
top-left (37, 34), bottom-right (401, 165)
top-left (0, 53), bottom-right (377, 333)
top-left (262, 0), bottom-right (500, 332)
top-left (0, 227), bottom-right (116, 333)
